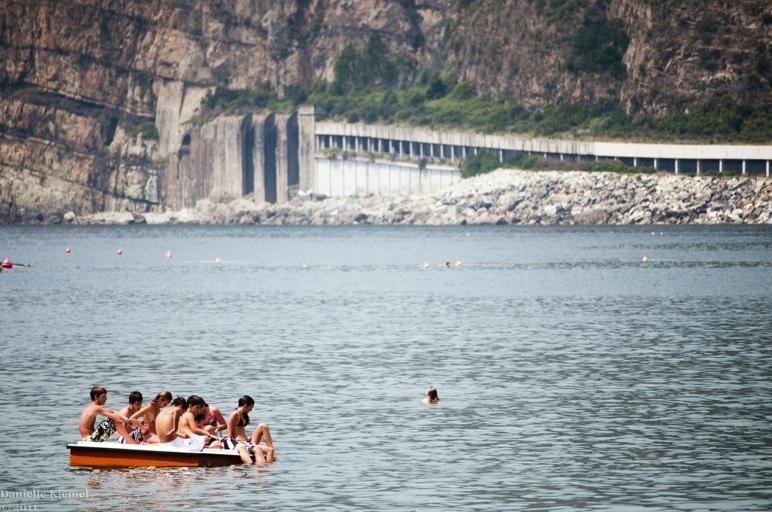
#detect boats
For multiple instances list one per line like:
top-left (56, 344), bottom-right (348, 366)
top-left (66, 441), bottom-right (274, 469)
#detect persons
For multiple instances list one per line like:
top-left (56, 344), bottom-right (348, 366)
top-left (425, 386), bottom-right (438, 404)
top-left (79, 386), bottom-right (275, 465)
